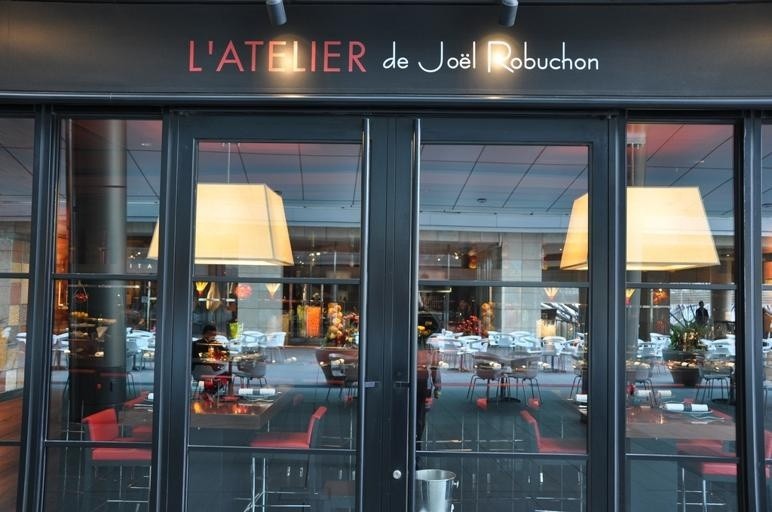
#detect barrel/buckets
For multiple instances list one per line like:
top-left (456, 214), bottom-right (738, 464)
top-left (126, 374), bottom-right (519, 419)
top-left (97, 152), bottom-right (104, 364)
top-left (415, 469), bottom-right (459, 511)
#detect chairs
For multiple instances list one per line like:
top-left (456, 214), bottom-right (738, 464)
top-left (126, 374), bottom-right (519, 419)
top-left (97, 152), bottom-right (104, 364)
top-left (14, 328), bottom-right (772, 404)
top-left (74, 388), bottom-right (350, 511)
top-left (424, 389), bottom-right (771, 509)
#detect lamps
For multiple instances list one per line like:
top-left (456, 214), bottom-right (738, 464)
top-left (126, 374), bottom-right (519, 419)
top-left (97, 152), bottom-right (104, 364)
top-left (558, 145), bottom-right (721, 270)
top-left (498, 1), bottom-right (518, 28)
top-left (267, 0), bottom-right (287, 25)
top-left (143, 143), bottom-right (294, 266)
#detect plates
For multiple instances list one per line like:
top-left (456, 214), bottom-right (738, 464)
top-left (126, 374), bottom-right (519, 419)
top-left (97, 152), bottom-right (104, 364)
top-left (235, 391), bottom-right (282, 399)
top-left (659, 404), bottom-right (713, 415)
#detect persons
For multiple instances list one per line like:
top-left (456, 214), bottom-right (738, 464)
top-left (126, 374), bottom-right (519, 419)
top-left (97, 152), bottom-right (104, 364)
top-left (191, 324), bottom-right (232, 405)
top-left (695, 300), bottom-right (710, 331)
top-left (416, 309), bottom-right (442, 471)
